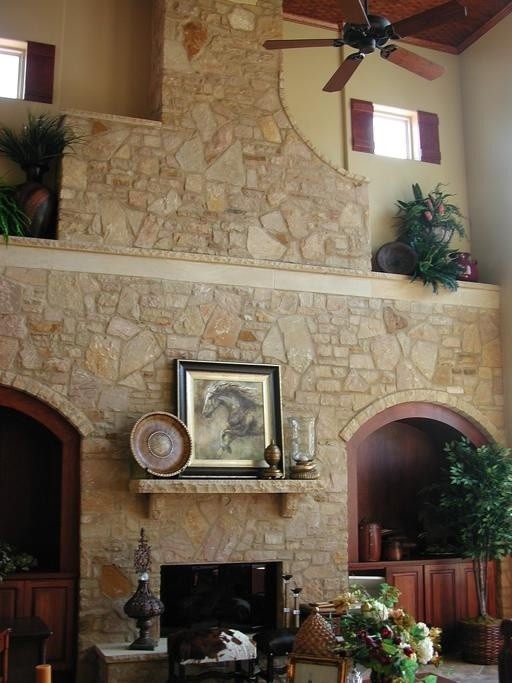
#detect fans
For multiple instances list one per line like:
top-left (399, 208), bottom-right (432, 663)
top-left (263, 1), bottom-right (468, 92)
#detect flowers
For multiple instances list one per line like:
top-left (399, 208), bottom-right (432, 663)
top-left (324, 582), bottom-right (444, 683)
top-left (390, 183), bottom-right (469, 238)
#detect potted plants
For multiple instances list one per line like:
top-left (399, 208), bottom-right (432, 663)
top-left (416, 434), bottom-right (512, 665)
top-left (0, 108), bottom-right (92, 238)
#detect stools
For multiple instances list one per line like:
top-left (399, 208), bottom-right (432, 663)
top-left (168, 627), bottom-right (259, 682)
top-left (249, 628), bottom-right (298, 683)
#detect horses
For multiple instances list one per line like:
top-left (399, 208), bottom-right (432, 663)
top-left (201, 380), bottom-right (264, 459)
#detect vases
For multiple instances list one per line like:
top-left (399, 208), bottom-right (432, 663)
top-left (376, 240), bottom-right (417, 274)
top-left (370, 670), bottom-right (384, 682)
top-left (398, 227), bottom-right (427, 245)
top-left (448, 251), bottom-right (479, 281)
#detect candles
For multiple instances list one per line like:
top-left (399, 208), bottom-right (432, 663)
top-left (34, 664), bottom-right (52, 681)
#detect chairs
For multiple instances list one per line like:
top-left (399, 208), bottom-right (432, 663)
top-left (0, 628), bottom-right (13, 683)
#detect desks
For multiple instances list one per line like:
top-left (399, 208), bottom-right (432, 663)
top-left (0, 616), bottom-right (52, 664)
top-left (94, 637), bottom-right (290, 682)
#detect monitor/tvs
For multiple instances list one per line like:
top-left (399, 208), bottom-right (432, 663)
top-left (159, 560), bottom-right (285, 655)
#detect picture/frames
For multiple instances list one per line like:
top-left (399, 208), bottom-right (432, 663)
top-left (286, 653), bottom-right (347, 682)
top-left (176, 359), bottom-right (286, 480)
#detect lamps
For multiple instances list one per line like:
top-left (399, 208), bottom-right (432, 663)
top-left (123, 527), bottom-right (166, 651)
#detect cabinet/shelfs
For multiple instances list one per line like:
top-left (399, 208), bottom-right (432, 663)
top-left (423, 558), bottom-right (497, 652)
top-left (0, 572), bottom-right (78, 672)
top-left (384, 561), bottom-right (424, 622)
